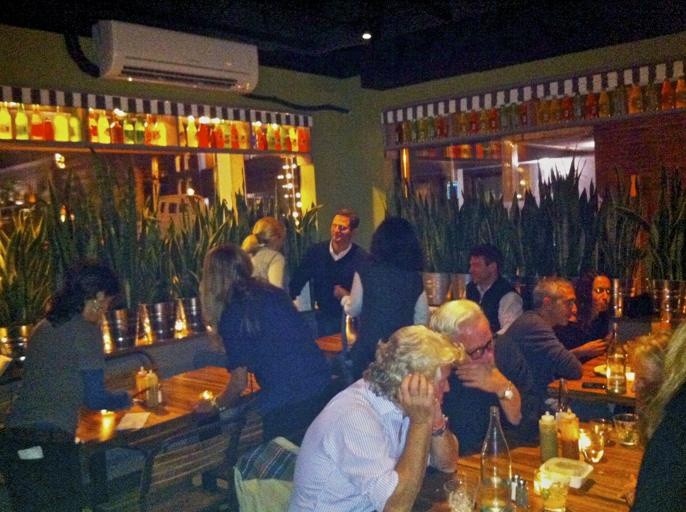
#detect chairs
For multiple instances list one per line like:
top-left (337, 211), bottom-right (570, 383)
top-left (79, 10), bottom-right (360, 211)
top-left (86, 349), bottom-right (190, 455)
top-left (90, 414), bottom-right (246, 511)
top-left (220, 390), bottom-right (265, 465)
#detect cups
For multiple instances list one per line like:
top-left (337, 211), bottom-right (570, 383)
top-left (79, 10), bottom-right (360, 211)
top-left (613, 413), bottom-right (640, 445)
top-left (587, 418), bottom-right (611, 442)
top-left (580, 433), bottom-right (606, 462)
top-left (537, 471), bottom-right (572, 512)
top-left (445, 478), bottom-right (480, 512)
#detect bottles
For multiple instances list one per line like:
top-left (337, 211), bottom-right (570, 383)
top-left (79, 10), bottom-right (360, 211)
top-left (460, 111), bottom-right (469, 136)
top-left (134, 115), bottom-right (146, 145)
top-left (560, 93), bottom-right (572, 121)
top-left (284, 126), bottom-right (292, 151)
top-left (549, 95), bottom-right (562, 121)
top-left (230, 124), bottom-right (240, 149)
top-left (210, 126), bottom-right (217, 148)
top-left (468, 108), bottom-right (479, 136)
top-left (143, 369), bottom-right (159, 408)
top-left (88, 107), bottom-right (99, 143)
top-left (31, 104), bottom-right (43, 141)
top-left (599, 84), bottom-right (611, 118)
top-left (572, 90), bottom-right (584, 120)
top-left (480, 406), bottom-right (512, 512)
top-left (42, 105), bottom-right (54, 141)
top-left (109, 112), bottom-right (123, 144)
top-left (274, 125), bottom-right (281, 150)
top-left (605, 322), bottom-right (626, 396)
top-left (178, 115), bottom-right (186, 146)
top-left (67, 108), bottom-right (81, 142)
top-left (135, 365), bottom-right (147, 401)
top-left (628, 78), bottom-right (643, 113)
top-left (53, 106), bottom-right (69, 142)
top-left (539, 411), bottom-right (558, 464)
top-left (488, 105), bottom-right (500, 133)
top-left (511, 102), bottom-right (520, 130)
top-left (479, 108), bottom-right (490, 134)
top-left (144, 114), bottom-right (154, 144)
top-left (393, 122), bottom-right (402, 146)
top-left (153, 115), bottom-right (167, 146)
top-left (509, 472), bottom-right (523, 501)
top-left (213, 122), bottom-right (225, 149)
top-left (402, 118), bottom-right (411, 142)
top-left (298, 127), bottom-right (310, 152)
top-left (289, 127), bottom-right (297, 151)
top-left (279, 126), bottom-right (286, 150)
top-left (433, 114), bottom-right (444, 139)
top-left (97, 109), bottom-right (110, 144)
top-left (186, 115), bottom-right (199, 147)
top-left (426, 115), bottom-right (436, 140)
top-left (558, 408), bottom-right (579, 463)
top-left (382, 123), bottom-right (392, 147)
top-left (14, 103), bottom-right (29, 140)
top-left (610, 82), bottom-right (627, 116)
top-left (197, 117), bottom-right (209, 148)
top-left (583, 87), bottom-right (598, 119)
top-left (266, 124), bottom-right (276, 150)
top-left (675, 76), bottom-right (686, 109)
top-left (499, 105), bottom-right (509, 131)
top-left (555, 408), bottom-right (565, 424)
top-left (536, 97), bottom-right (550, 125)
top-left (645, 77), bottom-right (660, 112)
top-left (417, 117), bottom-right (428, 139)
top-left (409, 112), bottom-right (418, 143)
top-left (257, 123), bottom-right (268, 151)
top-left (122, 114), bottom-right (134, 145)
top-left (516, 478), bottom-right (530, 509)
top-left (0, 101), bottom-right (13, 140)
top-left (661, 75), bottom-right (675, 110)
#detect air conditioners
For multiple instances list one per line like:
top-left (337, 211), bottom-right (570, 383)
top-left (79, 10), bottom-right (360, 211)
top-left (79, 21), bottom-right (259, 96)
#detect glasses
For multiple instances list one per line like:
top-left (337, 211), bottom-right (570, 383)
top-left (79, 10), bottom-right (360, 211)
top-left (590, 285), bottom-right (614, 296)
top-left (325, 222), bottom-right (354, 233)
top-left (456, 338), bottom-right (499, 361)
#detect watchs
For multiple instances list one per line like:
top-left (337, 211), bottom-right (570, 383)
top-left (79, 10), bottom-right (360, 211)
top-left (431, 414), bottom-right (451, 437)
top-left (210, 396), bottom-right (228, 414)
top-left (497, 380), bottom-right (513, 402)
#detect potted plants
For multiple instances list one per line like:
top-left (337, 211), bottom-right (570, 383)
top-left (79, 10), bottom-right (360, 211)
top-left (527, 191), bottom-right (561, 306)
top-left (420, 199), bottom-right (450, 307)
top-left (606, 179), bottom-right (641, 320)
top-left (568, 187), bottom-right (599, 283)
top-left (100, 160), bottom-right (140, 349)
top-left (449, 200), bottom-right (478, 301)
top-left (496, 200), bottom-right (528, 296)
top-left (139, 222), bottom-right (178, 341)
top-left (0, 218), bottom-right (53, 361)
top-left (645, 168), bottom-right (685, 329)
top-left (180, 220), bottom-right (217, 330)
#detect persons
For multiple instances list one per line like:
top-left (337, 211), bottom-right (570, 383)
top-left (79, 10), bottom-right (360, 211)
top-left (619, 321), bottom-right (686, 512)
top-left (333, 215), bottom-right (431, 381)
top-left (241, 215), bottom-right (300, 311)
top-left (286, 325), bottom-right (466, 512)
top-left (289, 208), bottom-right (371, 338)
top-left (0, 258), bottom-right (135, 512)
top-left (499, 275), bottom-right (613, 423)
top-left (193, 244), bottom-right (333, 494)
top-left (428, 298), bottom-right (559, 457)
top-left (464, 242), bottom-right (524, 336)
top-left (553, 270), bottom-right (635, 413)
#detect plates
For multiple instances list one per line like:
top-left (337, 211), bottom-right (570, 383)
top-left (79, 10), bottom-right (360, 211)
top-left (594, 363), bottom-right (635, 376)
